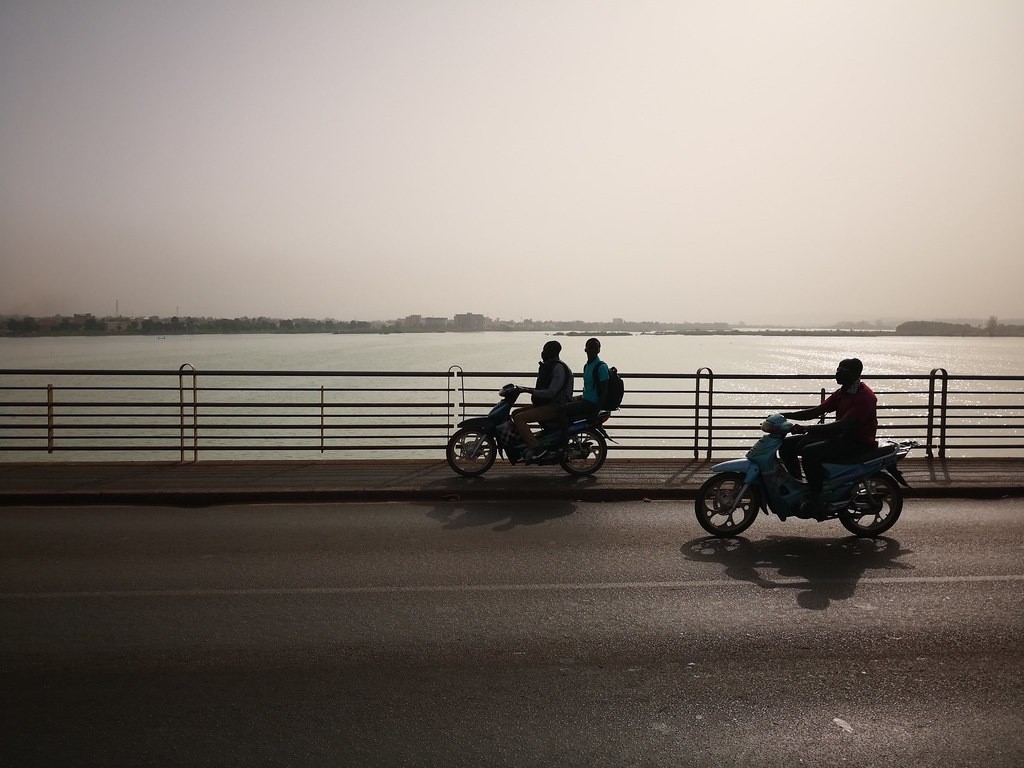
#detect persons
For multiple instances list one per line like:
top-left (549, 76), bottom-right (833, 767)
top-left (512, 338), bottom-right (624, 459)
top-left (767, 359), bottom-right (878, 511)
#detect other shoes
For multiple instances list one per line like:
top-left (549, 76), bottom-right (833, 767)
top-left (801, 498), bottom-right (823, 511)
top-left (525, 447), bottom-right (546, 466)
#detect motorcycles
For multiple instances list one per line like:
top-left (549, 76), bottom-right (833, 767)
top-left (695, 413), bottom-right (915, 535)
top-left (446, 383), bottom-right (618, 476)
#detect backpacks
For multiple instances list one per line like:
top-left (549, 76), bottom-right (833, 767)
top-left (584, 361), bottom-right (624, 411)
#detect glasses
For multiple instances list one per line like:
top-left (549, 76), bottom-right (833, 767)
top-left (837, 368), bottom-right (850, 373)
